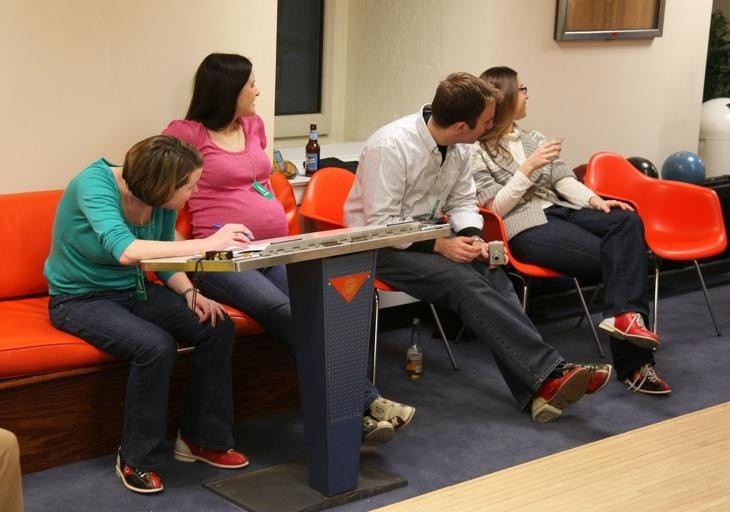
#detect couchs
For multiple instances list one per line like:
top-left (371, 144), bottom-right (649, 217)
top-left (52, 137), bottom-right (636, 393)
top-left (2, 170), bottom-right (301, 472)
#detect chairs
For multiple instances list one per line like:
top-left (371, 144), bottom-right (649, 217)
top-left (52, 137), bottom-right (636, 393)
top-left (576, 152), bottom-right (725, 357)
top-left (452, 207), bottom-right (609, 359)
top-left (296, 167), bottom-right (468, 387)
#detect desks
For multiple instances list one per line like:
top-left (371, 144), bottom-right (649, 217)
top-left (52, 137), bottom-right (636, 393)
top-left (366, 398), bottom-right (725, 509)
top-left (140, 222), bottom-right (452, 497)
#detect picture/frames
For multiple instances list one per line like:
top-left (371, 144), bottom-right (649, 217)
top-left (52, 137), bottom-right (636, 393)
top-left (555, 0), bottom-right (666, 42)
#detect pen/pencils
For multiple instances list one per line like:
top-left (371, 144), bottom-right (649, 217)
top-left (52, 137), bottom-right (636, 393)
top-left (211, 223), bottom-right (249, 239)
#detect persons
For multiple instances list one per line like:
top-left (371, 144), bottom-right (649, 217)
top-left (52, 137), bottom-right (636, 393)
top-left (44, 135), bottom-right (250, 493)
top-left (344, 72), bottom-right (613, 423)
top-left (161, 53), bottom-right (416, 454)
top-left (471, 66), bottom-right (671, 394)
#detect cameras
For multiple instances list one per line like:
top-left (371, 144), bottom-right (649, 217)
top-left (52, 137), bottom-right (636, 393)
top-left (489, 240), bottom-right (505, 265)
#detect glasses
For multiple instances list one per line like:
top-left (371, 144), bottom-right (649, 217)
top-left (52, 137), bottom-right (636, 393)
top-left (515, 86), bottom-right (528, 94)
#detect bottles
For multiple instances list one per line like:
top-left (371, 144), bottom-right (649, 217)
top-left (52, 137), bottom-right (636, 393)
top-left (405, 317), bottom-right (423, 382)
top-left (305, 124), bottom-right (319, 178)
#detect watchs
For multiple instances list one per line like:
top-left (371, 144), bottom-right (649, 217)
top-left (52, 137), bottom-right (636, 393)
top-left (472, 237), bottom-right (485, 242)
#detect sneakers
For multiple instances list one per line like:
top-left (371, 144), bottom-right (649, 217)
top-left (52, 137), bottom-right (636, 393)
top-left (531, 366), bottom-right (591, 423)
top-left (359, 414), bottom-right (395, 457)
top-left (114, 444), bottom-right (165, 495)
top-left (624, 362), bottom-right (673, 396)
top-left (598, 311), bottom-right (662, 350)
top-left (172, 426), bottom-right (250, 469)
top-left (549, 360), bottom-right (613, 395)
top-left (368, 395), bottom-right (416, 431)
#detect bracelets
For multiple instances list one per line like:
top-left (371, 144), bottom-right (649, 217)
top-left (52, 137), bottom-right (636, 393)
top-left (183, 287), bottom-right (200, 297)
